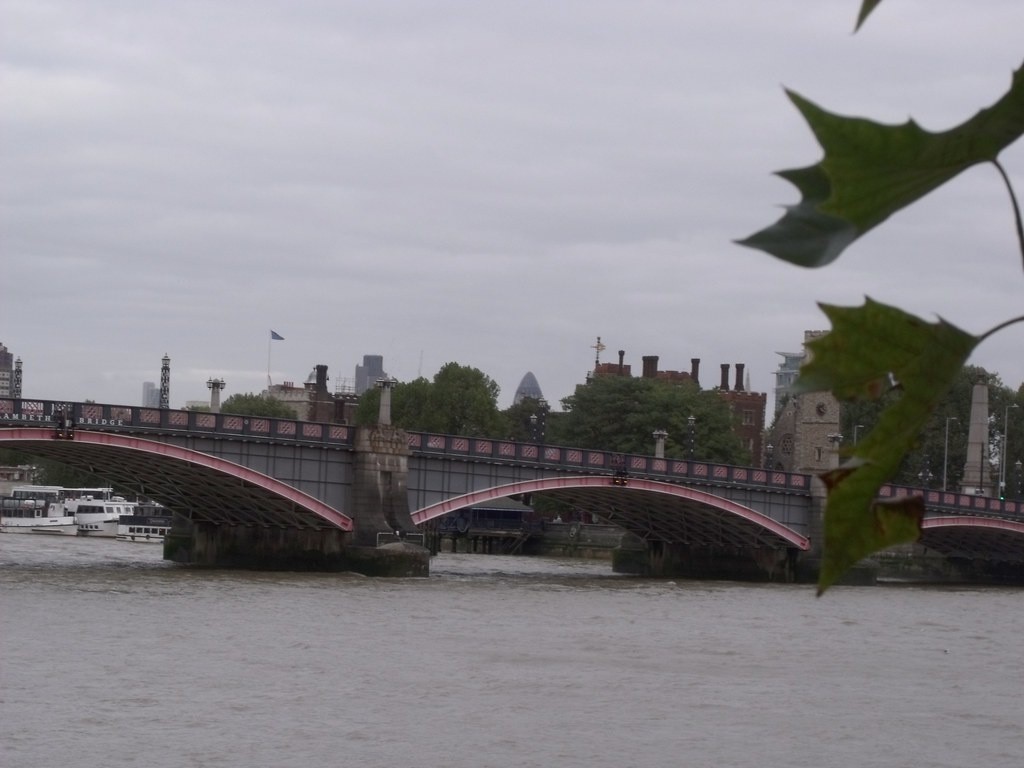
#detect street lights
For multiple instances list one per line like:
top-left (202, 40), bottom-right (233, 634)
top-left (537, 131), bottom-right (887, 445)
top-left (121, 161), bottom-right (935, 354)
top-left (1002, 405), bottom-right (1020, 498)
top-left (530, 414), bottom-right (538, 443)
top-left (687, 416), bottom-right (697, 461)
top-left (12, 358), bottom-right (23, 399)
top-left (766, 444), bottom-right (774, 487)
top-left (942, 417), bottom-right (958, 492)
top-left (160, 352), bottom-right (171, 408)
top-left (997, 434), bottom-right (1005, 500)
top-left (538, 397), bottom-right (547, 444)
top-left (853, 425), bottom-right (864, 449)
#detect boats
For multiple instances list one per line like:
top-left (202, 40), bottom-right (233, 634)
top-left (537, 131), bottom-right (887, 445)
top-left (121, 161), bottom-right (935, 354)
top-left (134, 507), bottom-right (136, 508)
top-left (0, 484), bottom-right (174, 544)
